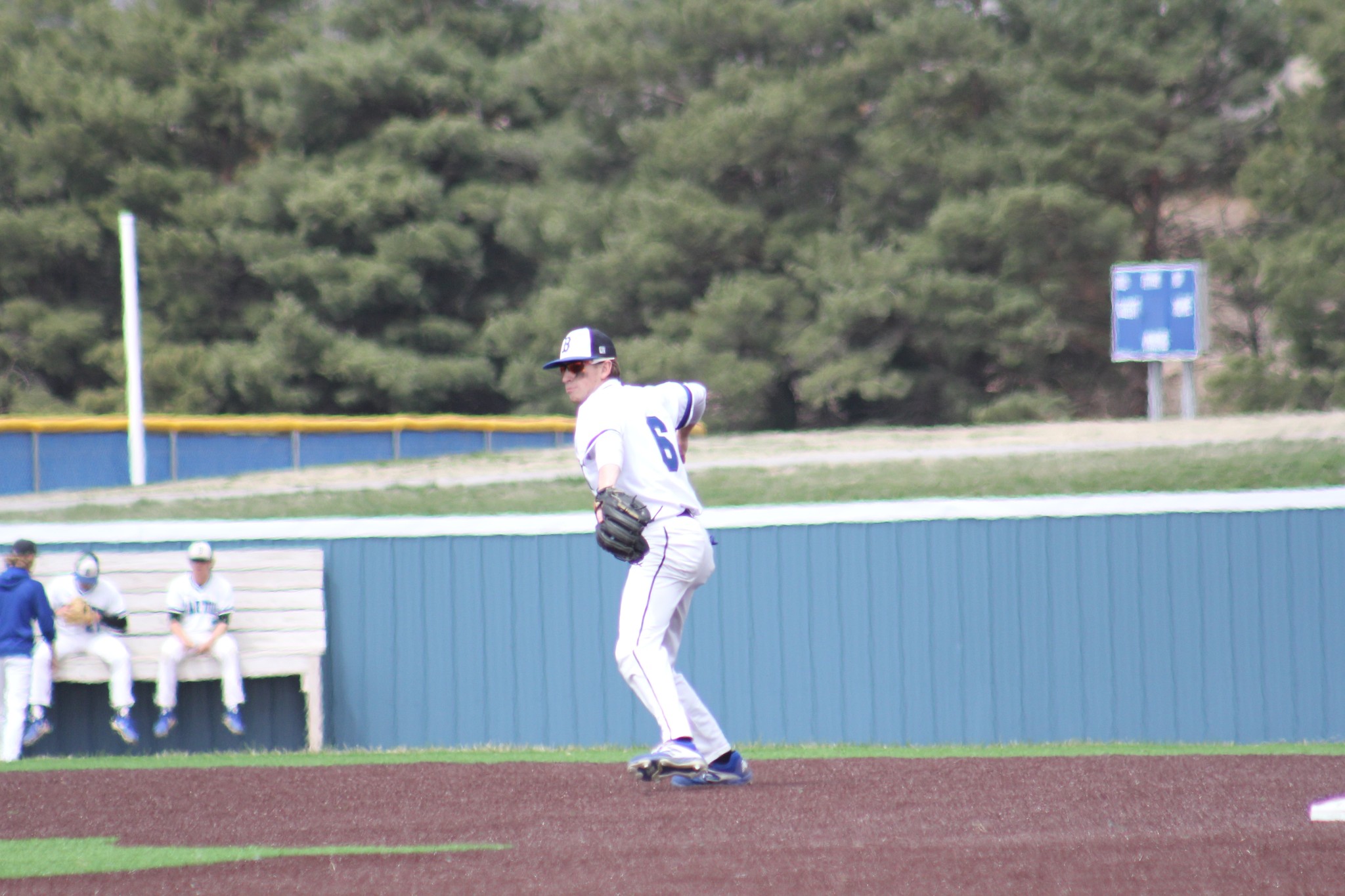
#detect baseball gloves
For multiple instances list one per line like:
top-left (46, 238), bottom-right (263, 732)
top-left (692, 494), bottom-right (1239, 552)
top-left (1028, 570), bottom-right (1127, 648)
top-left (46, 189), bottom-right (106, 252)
top-left (593, 490), bottom-right (651, 565)
top-left (64, 598), bottom-right (97, 627)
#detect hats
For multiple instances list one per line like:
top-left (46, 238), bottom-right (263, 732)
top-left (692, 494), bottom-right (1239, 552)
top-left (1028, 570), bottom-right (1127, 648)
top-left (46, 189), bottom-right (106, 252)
top-left (13, 540), bottom-right (38, 556)
top-left (543, 329), bottom-right (617, 369)
top-left (187, 542), bottom-right (212, 561)
top-left (72, 553), bottom-right (99, 584)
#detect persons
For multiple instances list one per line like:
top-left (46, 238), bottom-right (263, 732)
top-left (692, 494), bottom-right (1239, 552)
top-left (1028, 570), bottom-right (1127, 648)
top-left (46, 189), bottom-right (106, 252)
top-left (543, 327), bottom-right (751, 790)
top-left (0, 539), bottom-right (58, 765)
top-left (22, 552), bottom-right (139, 746)
top-left (152, 540), bottom-right (244, 736)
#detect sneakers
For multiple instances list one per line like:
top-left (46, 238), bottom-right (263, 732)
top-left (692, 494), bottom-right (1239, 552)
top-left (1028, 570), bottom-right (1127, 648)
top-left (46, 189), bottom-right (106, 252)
top-left (24, 716), bottom-right (49, 745)
top-left (221, 712), bottom-right (244, 735)
top-left (671, 752), bottom-right (752, 786)
top-left (111, 711), bottom-right (138, 743)
top-left (628, 742), bottom-right (707, 781)
top-left (152, 710), bottom-right (175, 737)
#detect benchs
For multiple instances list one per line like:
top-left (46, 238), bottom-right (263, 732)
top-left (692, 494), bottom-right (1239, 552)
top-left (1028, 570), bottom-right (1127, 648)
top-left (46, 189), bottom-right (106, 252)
top-left (0, 551), bottom-right (328, 753)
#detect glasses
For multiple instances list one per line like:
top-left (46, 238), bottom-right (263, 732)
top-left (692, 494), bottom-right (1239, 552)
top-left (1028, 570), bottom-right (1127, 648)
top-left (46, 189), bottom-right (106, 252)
top-left (560, 357), bottom-right (615, 375)
